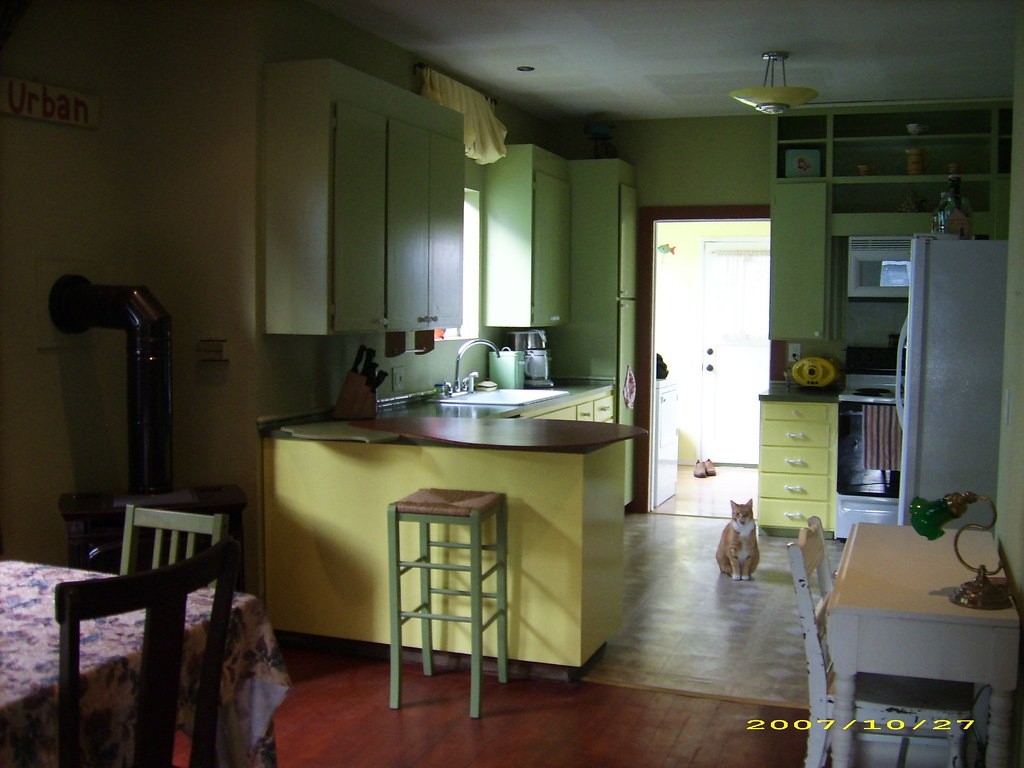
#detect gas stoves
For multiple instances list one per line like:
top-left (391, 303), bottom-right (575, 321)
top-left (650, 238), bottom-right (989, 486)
top-left (838, 345), bottom-right (905, 404)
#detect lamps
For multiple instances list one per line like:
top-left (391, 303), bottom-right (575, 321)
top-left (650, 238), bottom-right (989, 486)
top-left (909, 492), bottom-right (1011, 610)
top-left (729, 49), bottom-right (816, 114)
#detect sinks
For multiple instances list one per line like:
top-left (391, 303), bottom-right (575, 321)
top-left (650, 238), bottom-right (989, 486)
top-left (449, 388), bottom-right (565, 403)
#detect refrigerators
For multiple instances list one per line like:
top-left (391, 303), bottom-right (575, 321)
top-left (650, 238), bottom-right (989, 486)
top-left (894, 238), bottom-right (1008, 531)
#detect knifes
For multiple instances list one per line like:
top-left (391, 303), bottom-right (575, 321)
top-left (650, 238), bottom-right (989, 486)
top-left (350, 345), bottom-right (388, 390)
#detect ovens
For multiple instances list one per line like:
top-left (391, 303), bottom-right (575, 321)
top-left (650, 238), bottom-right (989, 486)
top-left (836, 400), bottom-right (904, 538)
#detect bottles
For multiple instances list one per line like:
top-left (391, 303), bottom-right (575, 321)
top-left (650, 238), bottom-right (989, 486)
top-left (905, 148), bottom-right (922, 174)
top-left (434, 384), bottom-right (444, 398)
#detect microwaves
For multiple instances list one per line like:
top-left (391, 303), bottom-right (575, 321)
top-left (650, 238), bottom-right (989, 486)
top-left (846, 235), bottom-right (911, 297)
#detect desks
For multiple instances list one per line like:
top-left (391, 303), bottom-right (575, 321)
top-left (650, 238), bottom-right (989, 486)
top-left (826, 523), bottom-right (1021, 768)
top-left (0, 559), bottom-right (291, 768)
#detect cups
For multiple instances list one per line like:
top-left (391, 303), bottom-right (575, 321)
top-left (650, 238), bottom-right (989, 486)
top-left (946, 160), bottom-right (960, 173)
top-left (858, 164), bottom-right (871, 176)
top-left (906, 123), bottom-right (929, 134)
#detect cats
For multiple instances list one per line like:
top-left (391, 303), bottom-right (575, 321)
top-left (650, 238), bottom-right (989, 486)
top-left (716, 499), bottom-right (760, 581)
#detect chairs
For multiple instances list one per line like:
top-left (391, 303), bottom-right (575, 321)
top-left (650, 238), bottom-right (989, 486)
top-left (117, 505), bottom-right (229, 590)
top-left (786, 516), bottom-right (971, 768)
top-left (54, 535), bottom-right (242, 768)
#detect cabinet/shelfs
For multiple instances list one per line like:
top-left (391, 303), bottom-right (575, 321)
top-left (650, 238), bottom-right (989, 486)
top-left (519, 394), bottom-right (613, 425)
top-left (547, 159), bottom-right (639, 507)
top-left (768, 105), bottom-right (1014, 343)
top-left (758, 399), bottom-right (839, 542)
top-left (265, 59), bottom-right (463, 337)
top-left (483, 143), bottom-right (572, 329)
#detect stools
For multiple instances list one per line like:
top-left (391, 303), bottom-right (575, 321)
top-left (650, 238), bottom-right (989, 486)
top-left (386, 488), bottom-right (508, 718)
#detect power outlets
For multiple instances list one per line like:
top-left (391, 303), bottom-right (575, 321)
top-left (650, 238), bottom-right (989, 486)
top-left (788, 343), bottom-right (801, 362)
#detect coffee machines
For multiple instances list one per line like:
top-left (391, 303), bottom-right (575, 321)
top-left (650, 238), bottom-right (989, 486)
top-left (507, 330), bottom-right (554, 388)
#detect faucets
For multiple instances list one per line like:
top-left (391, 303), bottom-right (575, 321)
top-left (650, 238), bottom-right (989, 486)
top-left (453, 339), bottom-right (500, 390)
